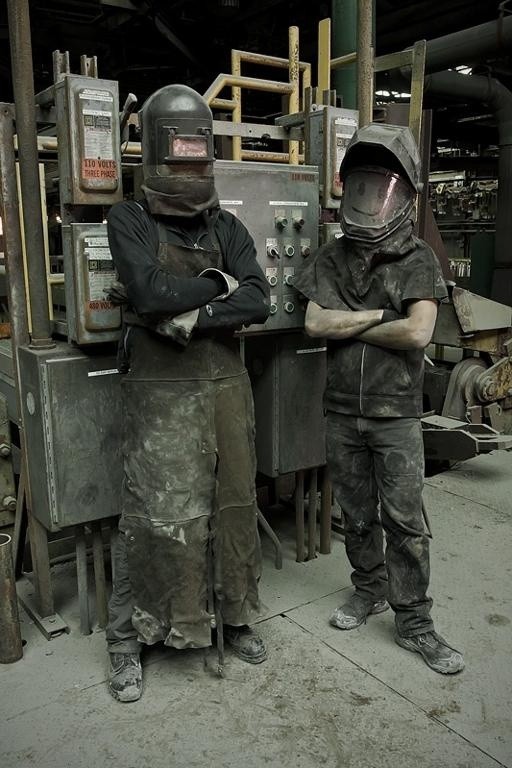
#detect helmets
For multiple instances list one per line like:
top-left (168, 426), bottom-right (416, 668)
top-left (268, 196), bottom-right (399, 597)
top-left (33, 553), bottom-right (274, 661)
top-left (141, 83), bottom-right (219, 218)
top-left (338, 121), bottom-right (424, 243)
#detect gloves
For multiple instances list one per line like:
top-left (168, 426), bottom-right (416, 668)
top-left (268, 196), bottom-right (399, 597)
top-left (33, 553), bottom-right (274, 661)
top-left (198, 267), bottom-right (239, 301)
top-left (103, 282), bottom-right (200, 347)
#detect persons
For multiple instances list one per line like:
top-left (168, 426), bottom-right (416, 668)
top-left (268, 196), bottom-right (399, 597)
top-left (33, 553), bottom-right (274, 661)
top-left (289, 123), bottom-right (466, 674)
top-left (104, 83), bottom-right (271, 702)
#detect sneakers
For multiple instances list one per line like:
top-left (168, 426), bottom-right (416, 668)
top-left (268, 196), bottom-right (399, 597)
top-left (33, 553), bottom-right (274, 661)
top-left (108, 653), bottom-right (142, 703)
top-left (223, 625), bottom-right (267, 664)
top-left (329, 592), bottom-right (390, 630)
top-left (395, 630), bottom-right (465, 675)
